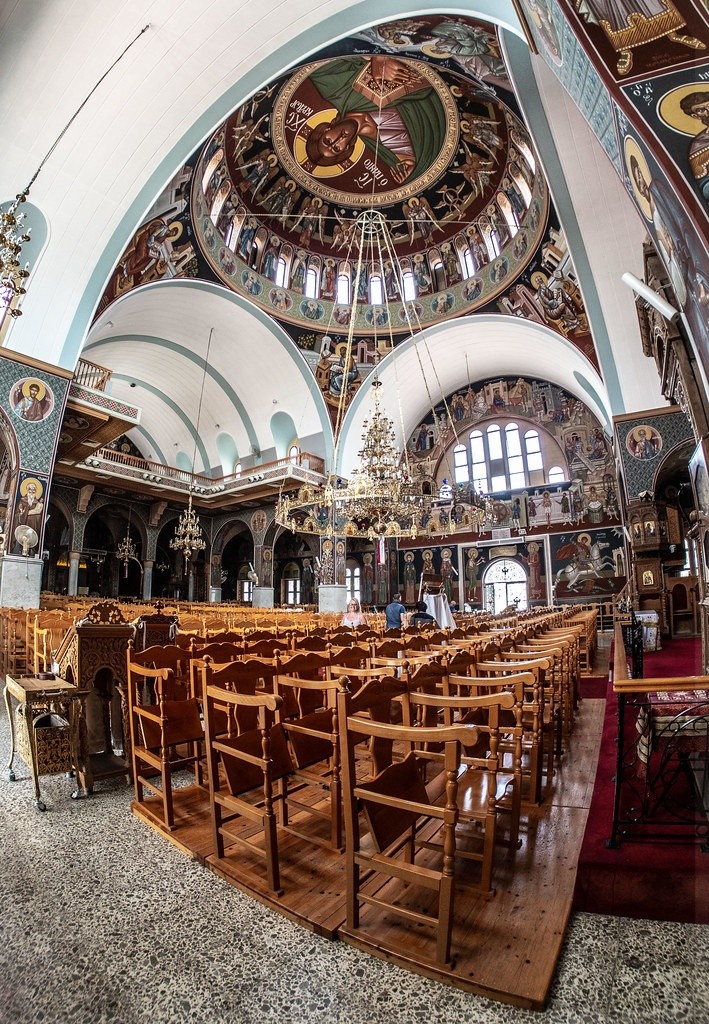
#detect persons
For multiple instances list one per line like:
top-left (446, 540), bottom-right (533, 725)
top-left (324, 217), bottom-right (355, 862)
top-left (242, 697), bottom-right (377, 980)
top-left (341, 598), bottom-right (367, 635)
top-left (410, 602), bottom-right (441, 629)
top-left (385, 593), bottom-right (405, 629)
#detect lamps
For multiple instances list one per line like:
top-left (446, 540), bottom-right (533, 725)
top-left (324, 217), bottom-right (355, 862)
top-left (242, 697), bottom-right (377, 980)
top-left (170, 329), bottom-right (214, 559)
top-left (274, 210), bottom-right (494, 541)
top-left (0, 24), bottom-right (152, 319)
top-left (115, 504), bottom-right (139, 563)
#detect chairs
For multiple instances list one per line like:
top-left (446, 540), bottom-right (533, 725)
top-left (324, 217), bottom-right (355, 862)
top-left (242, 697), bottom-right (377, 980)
top-left (3, 577), bottom-right (601, 967)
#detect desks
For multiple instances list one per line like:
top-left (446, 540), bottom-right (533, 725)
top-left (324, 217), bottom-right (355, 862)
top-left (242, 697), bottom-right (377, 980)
top-left (646, 691), bottom-right (708, 822)
top-left (635, 610), bottom-right (663, 651)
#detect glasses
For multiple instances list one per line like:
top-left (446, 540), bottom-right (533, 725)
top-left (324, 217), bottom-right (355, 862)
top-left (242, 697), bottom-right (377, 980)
top-left (349, 603), bottom-right (357, 606)
